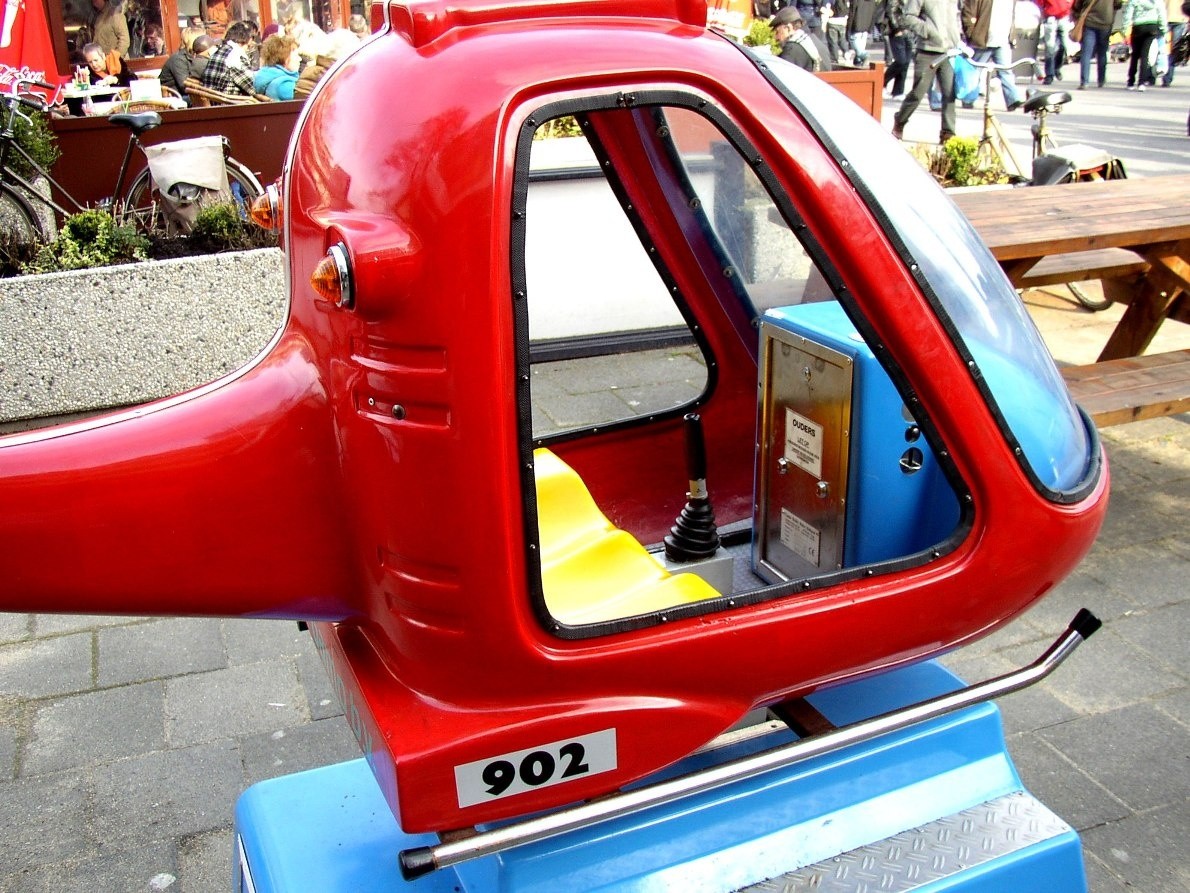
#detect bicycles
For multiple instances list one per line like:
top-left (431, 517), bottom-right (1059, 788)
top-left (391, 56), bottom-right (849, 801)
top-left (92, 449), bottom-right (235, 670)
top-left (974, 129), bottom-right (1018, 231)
top-left (929, 49), bottom-right (1134, 312)
top-left (0, 78), bottom-right (269, 260)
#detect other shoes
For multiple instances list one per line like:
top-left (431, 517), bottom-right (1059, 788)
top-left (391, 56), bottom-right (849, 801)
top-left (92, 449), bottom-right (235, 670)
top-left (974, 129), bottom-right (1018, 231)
top-left (1078, 84), bottom-right (1088, 89)
top-left (1162, 83), bottom-right (1170, 87)
top-left (963, 100), bottom-right (974, 108)
top-left (893, 94), bottom-right (906, 101)
top-left (939, 134), bottom-right (953, 144)
top-left (1008, 101), bottom-right (1026, 111)
top-left (1138, 84), bottom-right (1145, 91)
top-left (1125, 86), bottom-right (1133, 91)
top-left (1044, 77), bottom-right (1053, 85)
top-left (882, 88), bottom-right (893, 99)
top-left (1055, 72), bottom-right (1062, 81)
top-left (893, 117), bottom-right (904, 139)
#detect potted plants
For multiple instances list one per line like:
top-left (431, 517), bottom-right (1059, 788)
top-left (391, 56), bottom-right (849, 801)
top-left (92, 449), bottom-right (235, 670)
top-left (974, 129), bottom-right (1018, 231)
top-left (0, 188), bottom-right (287, 424)
top-left (0, 97), bottom-right (63, 243)
top-left (731, 131), bottom-right (1033, 284)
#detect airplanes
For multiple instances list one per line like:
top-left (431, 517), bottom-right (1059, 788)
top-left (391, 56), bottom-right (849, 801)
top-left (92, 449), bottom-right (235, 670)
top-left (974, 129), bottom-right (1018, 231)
top-left (0, 0), bottom-right (1113, 880)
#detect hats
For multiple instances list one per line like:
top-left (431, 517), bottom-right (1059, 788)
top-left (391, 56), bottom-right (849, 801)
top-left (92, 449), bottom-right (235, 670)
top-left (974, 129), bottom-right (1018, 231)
top-left (193, 35), bottom-right (219, 54)
top-left (769, 6), bottom-right (801, 27)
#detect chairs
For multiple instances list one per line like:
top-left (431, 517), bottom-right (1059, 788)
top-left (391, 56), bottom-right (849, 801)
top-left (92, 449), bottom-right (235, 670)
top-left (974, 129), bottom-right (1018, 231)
top-left (105, 74), bottom-right (276, 117)
top-left (533, 447), bottom-right (722, 626)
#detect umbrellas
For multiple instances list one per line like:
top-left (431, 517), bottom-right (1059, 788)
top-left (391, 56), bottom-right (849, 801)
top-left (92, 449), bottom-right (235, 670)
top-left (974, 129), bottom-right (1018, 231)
top-left (1, 0), bottom-right (65, 109)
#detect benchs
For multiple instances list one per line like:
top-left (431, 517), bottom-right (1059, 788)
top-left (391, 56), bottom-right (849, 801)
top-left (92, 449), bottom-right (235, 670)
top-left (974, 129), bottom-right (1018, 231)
top-left (1015, 247), bottom-right (1152, 289)
top-left (1058, 348), bottom-right (1190, 428)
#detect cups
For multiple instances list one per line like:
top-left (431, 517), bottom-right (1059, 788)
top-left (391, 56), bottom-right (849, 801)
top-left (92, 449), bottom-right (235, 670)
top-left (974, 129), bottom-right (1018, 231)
top-left (77, 84), bottom-right (88, 90)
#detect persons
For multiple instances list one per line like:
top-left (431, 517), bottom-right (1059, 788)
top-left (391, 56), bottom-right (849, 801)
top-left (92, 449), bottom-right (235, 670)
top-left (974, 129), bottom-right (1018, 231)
top-left (69, 0), bottom-right (369, 104)
top-left (762, 0), bottom-right (1190, 145)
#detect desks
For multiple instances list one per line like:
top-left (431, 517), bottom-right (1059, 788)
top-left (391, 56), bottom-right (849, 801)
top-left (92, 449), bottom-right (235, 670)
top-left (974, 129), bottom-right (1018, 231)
top-left (61, 85), bottom-right (187, 117)
top-left (766, 174), bottom-right (1190, 363)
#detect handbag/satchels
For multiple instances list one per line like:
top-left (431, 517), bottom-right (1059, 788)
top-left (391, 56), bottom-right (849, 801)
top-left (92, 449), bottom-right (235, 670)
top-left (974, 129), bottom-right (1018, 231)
top-left (144, 135), bottom-right (230, 236)
top-left (953, 54), bottom-right (980, 101)
top-left (1154, 37), bottom-right (1169, 77)
top-left (1068, 15), bottom-right (1085, 43)
